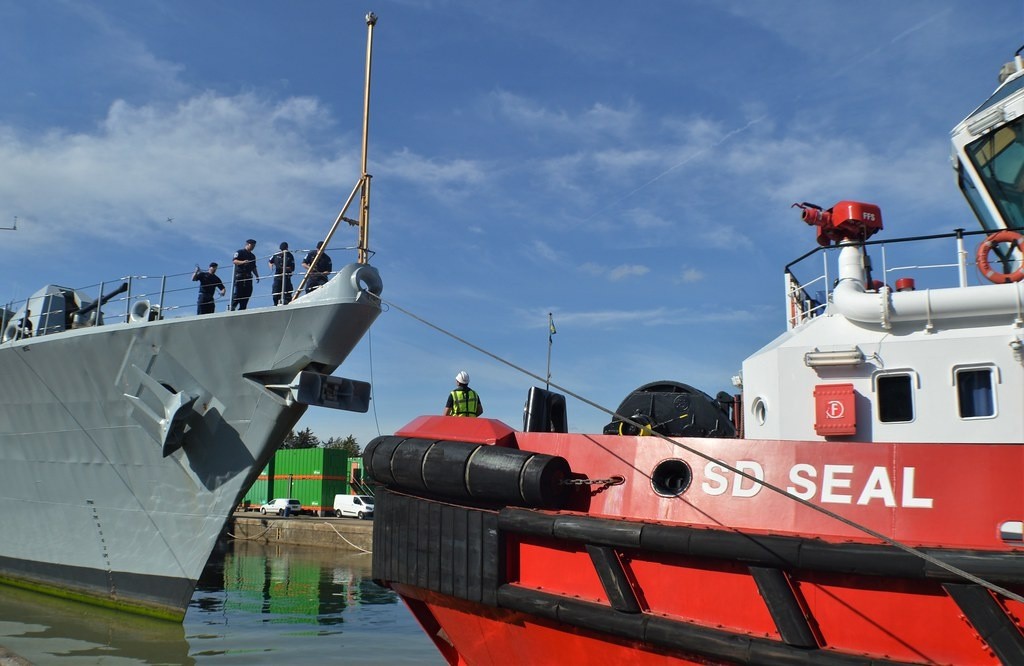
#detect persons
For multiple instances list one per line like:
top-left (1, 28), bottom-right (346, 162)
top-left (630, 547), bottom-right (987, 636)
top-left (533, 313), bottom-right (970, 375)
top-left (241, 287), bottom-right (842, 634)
top-left (190, 262), bottom-right (225, 315)
top-left (443, 371), bottom-right (483, 419)
top-left (268, 242), bottom-right (295, 307)
top-left (302, 241), bottom-right (332, 294)
top-left (16, 310), bottom-right (33, 341)
top-left (232, 239), bottom-right (261, 311)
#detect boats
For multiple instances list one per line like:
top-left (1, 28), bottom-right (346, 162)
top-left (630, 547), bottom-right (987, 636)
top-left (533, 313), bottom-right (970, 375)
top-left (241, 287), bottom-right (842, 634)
top-left (1, 24), bottom-right (385, 627)
top-left (358, 38), bottom-right (1023, 666)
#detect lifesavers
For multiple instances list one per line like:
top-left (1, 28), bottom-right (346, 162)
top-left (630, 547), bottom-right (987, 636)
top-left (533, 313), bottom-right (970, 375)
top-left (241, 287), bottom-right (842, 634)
top-left (977, 230), bottom-right (1024, 284)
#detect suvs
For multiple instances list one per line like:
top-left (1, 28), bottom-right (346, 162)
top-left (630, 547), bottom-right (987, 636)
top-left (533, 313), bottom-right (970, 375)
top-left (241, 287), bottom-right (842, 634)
top-left (260, 499), bottom-right (301, 517)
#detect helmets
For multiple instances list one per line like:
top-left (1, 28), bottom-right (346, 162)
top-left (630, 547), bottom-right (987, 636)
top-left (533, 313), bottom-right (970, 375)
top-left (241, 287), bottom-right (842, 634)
top-left (455, 371), bottom-right (470, 384)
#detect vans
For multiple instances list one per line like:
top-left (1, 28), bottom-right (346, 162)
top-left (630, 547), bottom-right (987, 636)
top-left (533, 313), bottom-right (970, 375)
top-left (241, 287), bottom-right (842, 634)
top-left (333, 494), bottom-right (375, 520)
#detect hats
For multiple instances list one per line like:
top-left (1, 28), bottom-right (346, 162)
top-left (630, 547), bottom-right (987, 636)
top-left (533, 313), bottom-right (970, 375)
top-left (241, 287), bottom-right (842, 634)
top-left (317, 242), bottom-right (323, 249)
top-left (246, 239), bottom-right (256, 244)
top-left (280, 242), bottom-right (288, 250)
top-left (210, 262), bottom-right (218, 267)
